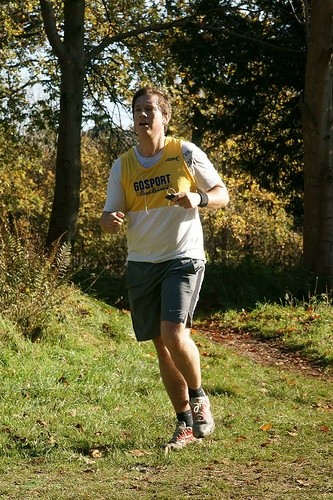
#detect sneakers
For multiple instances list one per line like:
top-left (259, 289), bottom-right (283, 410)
top-left (189, 395), bottom-right (215, 438)
top-left (168, 421), bottom-right (202, 449)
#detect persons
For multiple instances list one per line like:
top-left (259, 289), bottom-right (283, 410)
top-left (100, 88), bottom-right (229, 448)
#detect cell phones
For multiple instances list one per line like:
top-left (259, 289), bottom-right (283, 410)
top-left (165, 193), bottom-right (177, 201)
